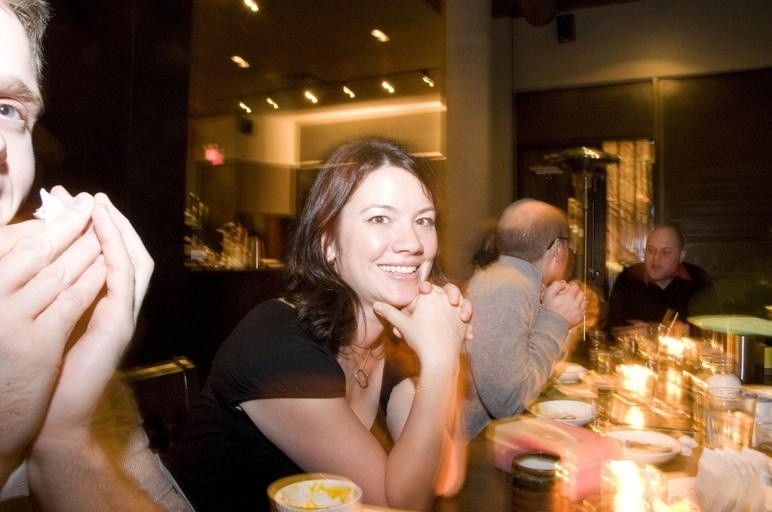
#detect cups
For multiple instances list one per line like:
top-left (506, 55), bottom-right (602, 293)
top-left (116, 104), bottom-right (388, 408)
top-left (274, 478), bottom-right (365, 511)
top-left (706, 387), bottom-right (758, 452)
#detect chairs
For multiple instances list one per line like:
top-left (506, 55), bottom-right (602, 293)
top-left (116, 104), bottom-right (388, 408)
top-left (122, 356), bottom-right (208, 506)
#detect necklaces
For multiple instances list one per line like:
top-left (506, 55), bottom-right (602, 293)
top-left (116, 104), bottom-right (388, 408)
top-left (346, 340), bottom-right (375, 393)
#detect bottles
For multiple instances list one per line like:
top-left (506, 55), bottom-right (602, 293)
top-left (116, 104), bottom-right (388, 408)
top-left (692, 349), bottom-right (741, 431)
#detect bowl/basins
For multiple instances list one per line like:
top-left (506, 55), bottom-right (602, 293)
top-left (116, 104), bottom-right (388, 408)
top-left (554, 363), bottom-right (588, 384)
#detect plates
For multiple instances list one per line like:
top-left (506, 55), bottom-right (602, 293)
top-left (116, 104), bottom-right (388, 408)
top-left (600, 429), bottom-right (683, 465)
top-left (530, 399), bottom-right (597, 426)
top-left (578, 369), bottom-right (617, 392)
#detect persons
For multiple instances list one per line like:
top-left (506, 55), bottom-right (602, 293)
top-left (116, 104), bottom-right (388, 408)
top-left (608, 221), bottom-right (718, 348)
top-left (465, 198), bottom-right (599, 446)
top-left (0, 0), bottom-right (197, 512)
top-left (183, 135), bottom-right (478, 512)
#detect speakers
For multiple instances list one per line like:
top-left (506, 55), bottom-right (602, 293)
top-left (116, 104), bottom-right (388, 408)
top-left (556, 13), bottom-right (577, 43)
